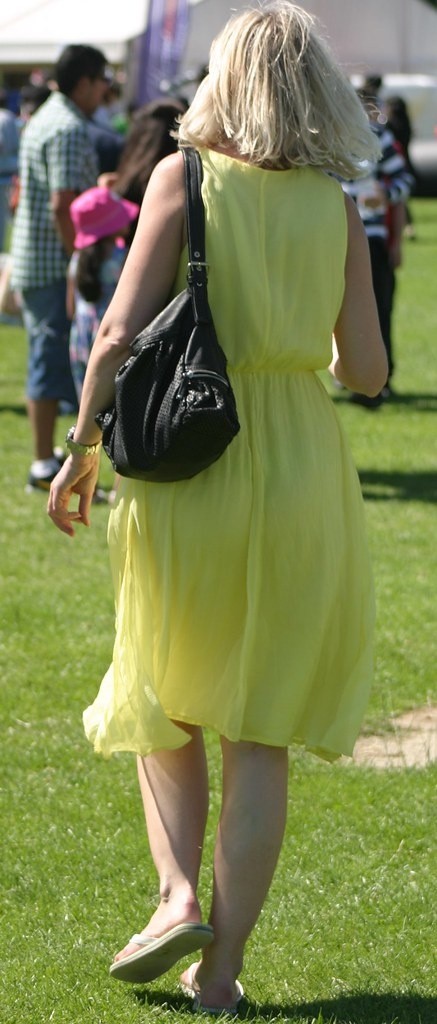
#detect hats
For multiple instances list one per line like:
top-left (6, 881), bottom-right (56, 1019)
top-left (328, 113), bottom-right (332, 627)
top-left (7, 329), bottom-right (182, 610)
top-left (347, 73), bottom-right (381, 99)
top-left (70, 185), bottom-right (139, 250)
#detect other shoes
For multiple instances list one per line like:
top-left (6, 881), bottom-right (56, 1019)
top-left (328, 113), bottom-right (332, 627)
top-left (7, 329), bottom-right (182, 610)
top-left (28, 451), bottom-right (106, 502)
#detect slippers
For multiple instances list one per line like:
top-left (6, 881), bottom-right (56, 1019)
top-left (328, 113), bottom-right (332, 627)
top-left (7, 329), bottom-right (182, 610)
top-left (178, 960), bottom-right (245, 1017)
top-left (108, 920), bottom-right (213, 983)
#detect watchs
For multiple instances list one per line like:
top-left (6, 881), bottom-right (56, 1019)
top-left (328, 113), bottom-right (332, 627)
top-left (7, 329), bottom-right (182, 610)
top-left (65, 424), bottom-right (102, 456)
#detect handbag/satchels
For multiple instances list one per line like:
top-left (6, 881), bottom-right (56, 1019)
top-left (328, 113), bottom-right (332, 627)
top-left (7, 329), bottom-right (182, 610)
top-left (94, 145), bottom-right (241, 484)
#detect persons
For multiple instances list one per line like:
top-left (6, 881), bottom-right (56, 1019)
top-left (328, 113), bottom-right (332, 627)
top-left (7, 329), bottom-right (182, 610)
top-left (1, 44), bottom-right (186, 507)
top-left (325, 74), bottom-right (416, 394)
top-left (46, 1), bottom-right (389, 1011)
